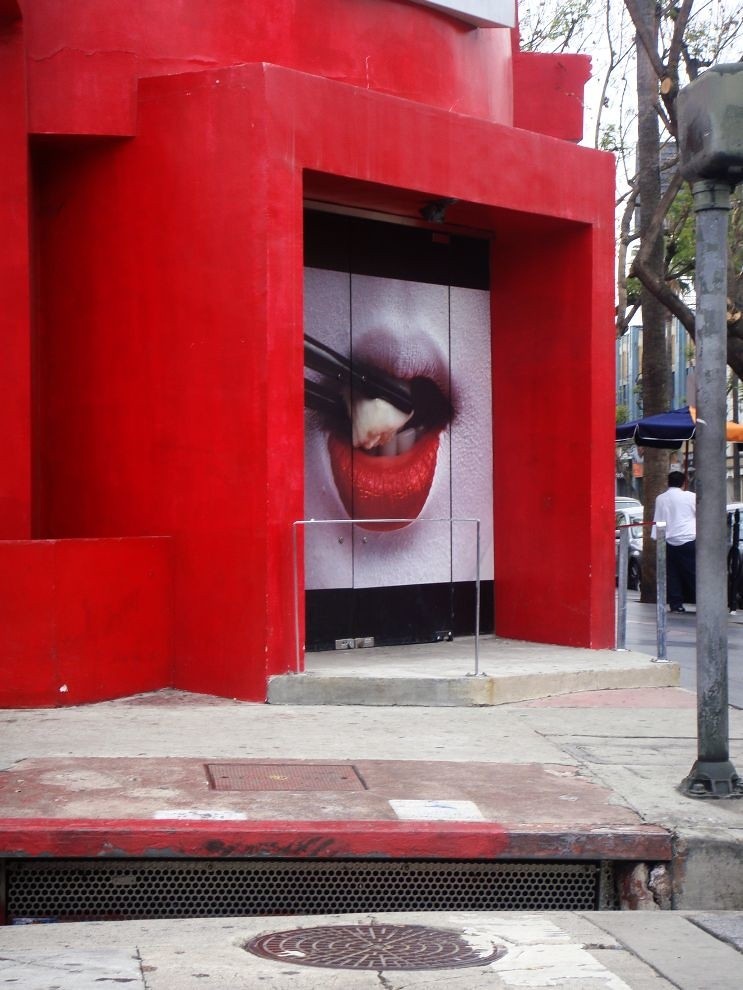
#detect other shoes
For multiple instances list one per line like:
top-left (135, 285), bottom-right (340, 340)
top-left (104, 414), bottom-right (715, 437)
top-left (670, 605), bottom-right (685, 613)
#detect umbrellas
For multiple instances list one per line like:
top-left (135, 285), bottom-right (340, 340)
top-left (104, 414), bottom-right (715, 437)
top-left (616, 406), bottom-right (743, 493)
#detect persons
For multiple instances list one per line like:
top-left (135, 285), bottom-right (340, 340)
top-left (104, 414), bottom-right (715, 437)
top-left (652, 471), bottom-right (697, 614)
top-left (305, 267), bottom-right (493, 589)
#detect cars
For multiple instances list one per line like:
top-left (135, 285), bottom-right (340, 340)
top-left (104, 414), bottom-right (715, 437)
top-left (615, 496), bottom-right (642, 510)
top-left (726, 502), bottom-right (743, 609)
top-left (615, 506), bottom-right (644, 592)
